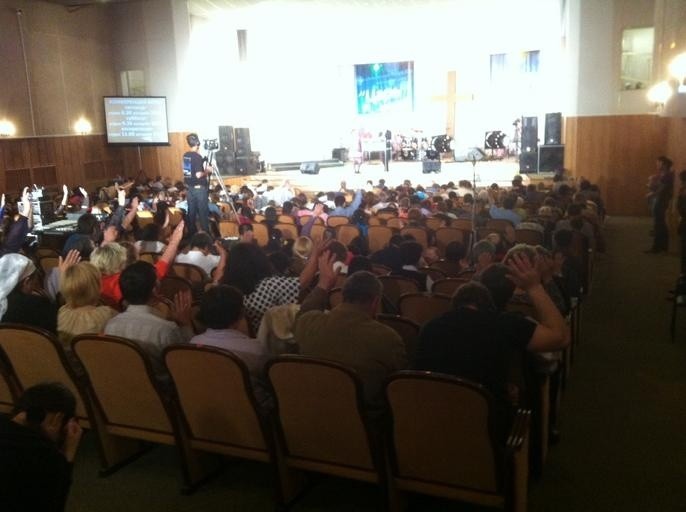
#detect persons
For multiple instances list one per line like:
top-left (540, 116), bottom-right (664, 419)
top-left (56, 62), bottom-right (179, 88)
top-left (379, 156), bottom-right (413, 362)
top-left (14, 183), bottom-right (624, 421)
top-left (183, 134), bottom-right (213, 233)
top-left (0, 172), bottom-right (605, 512)
top-left (347, 128), bottom-right (362, 173)
top-left (644, 157), bottom-right (673, 253)
top-left (676, 171), bottom-right (686, 285)
top-left (378, 125), bottom-right (392, 172)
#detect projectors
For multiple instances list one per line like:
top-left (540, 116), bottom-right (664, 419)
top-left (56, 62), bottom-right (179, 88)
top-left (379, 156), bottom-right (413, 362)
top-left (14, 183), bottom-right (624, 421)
top-left (21, 186), bottom-right (46, 201)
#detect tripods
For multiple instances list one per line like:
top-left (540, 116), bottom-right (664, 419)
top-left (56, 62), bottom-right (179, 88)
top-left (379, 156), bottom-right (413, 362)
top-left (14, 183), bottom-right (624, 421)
top-left (205, 151), bottom-right (240, 225)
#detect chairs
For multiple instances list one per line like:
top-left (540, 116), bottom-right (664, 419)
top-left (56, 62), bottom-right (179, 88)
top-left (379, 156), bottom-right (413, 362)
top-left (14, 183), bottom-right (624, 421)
top-left (69, 333), bottom-right (200, 495)
top-left (162, 343), bottom-right (297, 509)
top-left (1, 323), bottom-right (110, 471)
top-left (262, 354), bottom-right (390, 509)
top-left (537, 370), bottom-right (565, 479)
top-left (0, 181), bottom-right (598, 370)
top-left (383, 368), bottom-right (531, 510)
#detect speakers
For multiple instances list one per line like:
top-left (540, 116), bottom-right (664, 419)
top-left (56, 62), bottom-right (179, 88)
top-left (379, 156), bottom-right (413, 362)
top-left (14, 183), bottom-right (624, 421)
top-left (519, 112), bottom-right (565, 174)
top-left (423, 162), bottom-right (441, 173)
top-left (300, 162), bottom-right (319, 174)
top-left (215, 126), bottom-right (258, 175)
